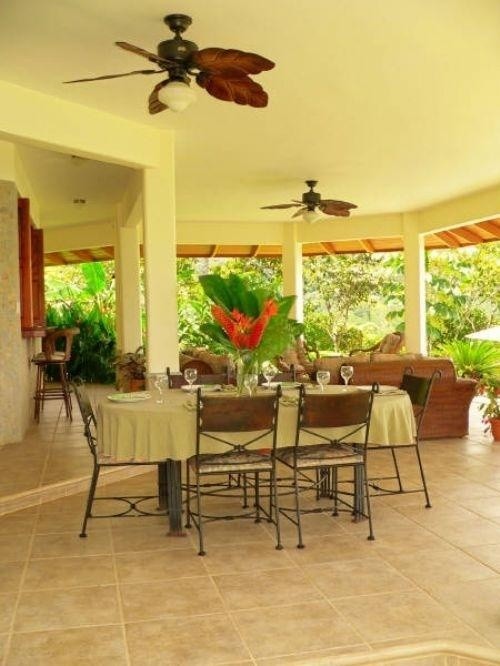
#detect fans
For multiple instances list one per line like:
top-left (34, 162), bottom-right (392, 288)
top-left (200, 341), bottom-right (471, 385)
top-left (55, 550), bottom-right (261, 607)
top-left (259, 178), bottom-right (359, 224)
top-left (61, 14), bottom-right (278, 116)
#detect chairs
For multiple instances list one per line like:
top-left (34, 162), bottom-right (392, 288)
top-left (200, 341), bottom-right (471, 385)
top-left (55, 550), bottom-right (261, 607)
top-left (30, 326), bottom-right (82, 424)
top-left (184, 385), bottom-right (284, 557)
top-left (164, 365), bottom-right (251, 512)
top-left (70, 376), bottom-right (202, 538)
top-left (252, 364), bottom-right (321, 509)
top-left (364, 365), bottom-right (444, 509)
top-left (265, 382), bottom-right (379, 549)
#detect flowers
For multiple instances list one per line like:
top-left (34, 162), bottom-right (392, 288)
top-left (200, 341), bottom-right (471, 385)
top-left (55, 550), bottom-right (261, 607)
top-left (196, 270), bottom-right (311, 392)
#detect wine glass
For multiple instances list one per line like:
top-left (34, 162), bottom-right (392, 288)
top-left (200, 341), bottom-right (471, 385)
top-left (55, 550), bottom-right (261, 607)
top-left (244, 373), bottom-right (258, 398)
top-left (183, 368), bottom-right (198, 395)
top-left (317, 371), bottom-right (331, 393)
top-left (263, 364), bottom-right (276, 394)
top-left (340, 366), bottom-right (354, 391)
top-left (153, 374), bottom-right (168, 405)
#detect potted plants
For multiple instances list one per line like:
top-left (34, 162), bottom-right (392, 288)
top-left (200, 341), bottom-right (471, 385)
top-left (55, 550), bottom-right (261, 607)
top-left (478, 372), bottom-right (500, 443)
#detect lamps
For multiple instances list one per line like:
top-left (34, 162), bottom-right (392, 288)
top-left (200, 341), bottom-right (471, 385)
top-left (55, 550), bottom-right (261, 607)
top-left (303, 208), bottom-right (324, 224)
top-left (158, 81), bottom-right (200, 113)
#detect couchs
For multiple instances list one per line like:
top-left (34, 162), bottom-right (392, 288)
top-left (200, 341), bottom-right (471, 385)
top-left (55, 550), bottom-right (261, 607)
top-left (117, 350), bottom-right (238, 393)
top-left (349, 333), bottom-right (404, 358)
top-left (313, 354), bottom-right (478, 440)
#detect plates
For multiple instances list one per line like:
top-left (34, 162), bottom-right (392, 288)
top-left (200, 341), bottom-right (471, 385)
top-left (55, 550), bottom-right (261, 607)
top-left (182, 385), bottom-right (203, 392)
top-left (107, 394), bottom-right (153, 403)
top-left (262, 382), bottom-right (304, 390)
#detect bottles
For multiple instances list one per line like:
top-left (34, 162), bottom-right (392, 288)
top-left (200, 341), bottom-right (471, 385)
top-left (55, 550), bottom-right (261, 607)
top-left (372, 381), bottom-right (380, 393)
